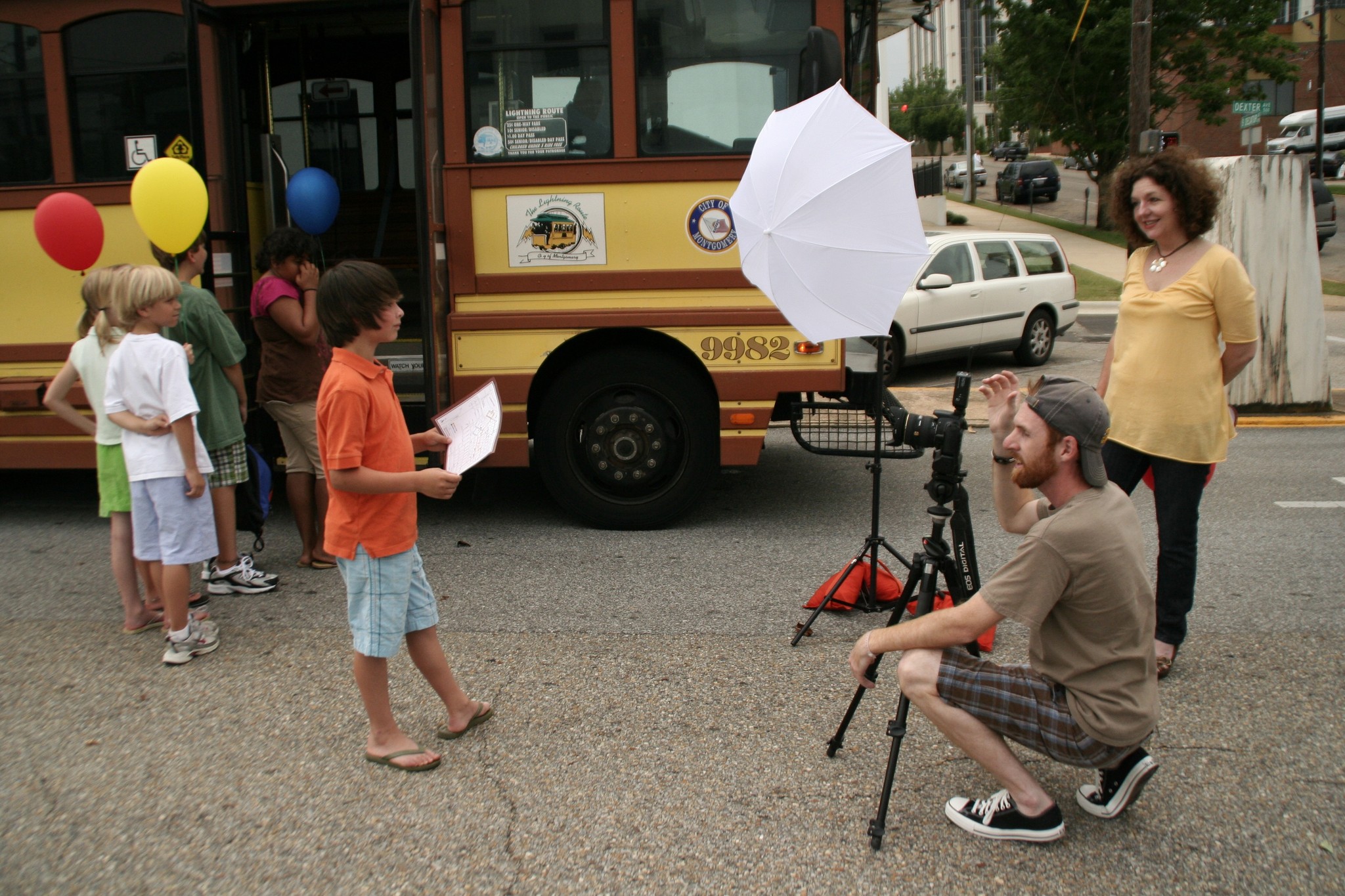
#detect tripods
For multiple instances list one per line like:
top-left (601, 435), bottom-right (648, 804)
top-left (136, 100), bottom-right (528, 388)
top-left (827, 481), bottom-right (982, 850)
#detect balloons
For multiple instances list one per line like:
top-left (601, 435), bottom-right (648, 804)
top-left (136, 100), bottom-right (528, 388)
top-left (34, 192), bottom-right (104, 276)
top-left (131, 158), bottom-right (209, 257)
top-left (286, 168), bottom-right (339, 235)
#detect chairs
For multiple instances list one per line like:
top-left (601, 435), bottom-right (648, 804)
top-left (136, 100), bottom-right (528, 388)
top-left (983, 253), bottom-right (1015, 280)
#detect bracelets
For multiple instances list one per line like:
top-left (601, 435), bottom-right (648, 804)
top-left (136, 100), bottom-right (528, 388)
top-left (991, 448), bottom-right (1016, 465)
top-left (303, 288), bottom-right (318, 295)
top-left (866, 630), bottom-right (877, 659)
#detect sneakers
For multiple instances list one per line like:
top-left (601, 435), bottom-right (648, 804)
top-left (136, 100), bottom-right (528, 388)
top-left (944, 788), bottom-right (1065, 841)
top-left (162, 631), bottom-right (220, 664)
top-left (207, 556), bottom-right (277, 594)
top-left (1077, 746), bottom-right (1159, 819)
top-left (188, 620), bottom-right (220, 634)
top-left (201, 559), bottom-right (211, 579)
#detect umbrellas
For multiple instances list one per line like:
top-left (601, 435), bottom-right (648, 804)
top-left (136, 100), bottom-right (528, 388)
top-left (729, 79), bottom-right (936, 345)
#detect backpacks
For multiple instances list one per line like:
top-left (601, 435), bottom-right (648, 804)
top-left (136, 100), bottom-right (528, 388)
top-left (235, 443), bottom-right (271, 551)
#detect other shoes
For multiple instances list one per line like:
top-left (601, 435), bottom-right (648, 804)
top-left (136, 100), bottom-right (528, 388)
top-left (1157, 644), bottom-right (1180, 678)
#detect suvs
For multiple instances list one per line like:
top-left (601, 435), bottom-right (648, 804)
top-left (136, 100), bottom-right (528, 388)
top-left (1310, 177), bottom-right (1339, 253)
top-left (995, 160), bottom-right (1061, 205)
top-left (846, 230), bottom-right (1083, 395)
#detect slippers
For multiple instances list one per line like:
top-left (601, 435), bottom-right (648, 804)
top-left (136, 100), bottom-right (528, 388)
top-left (312, 560), bottom-right (337, 568)
top-left (297, 561), bottom-right (309, 566)
top-left (438, 702), bottom-right (493, 739)
top-left (367, 743), bottom-right (441, 771)
top-left (124, 611), bottom-right (164, 634)
top-left (153, 592), bottom-right (210, 611)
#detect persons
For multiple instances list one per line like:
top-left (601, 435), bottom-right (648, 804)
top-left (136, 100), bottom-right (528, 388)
top-left (43, 263), bottom-right (210, 633)
top-left (314, 261), bottom-right (495, 774)
top-left (152, 229), bottom-right (283, 595)
top-left (251, 230), bottom-right (337, 568)
top-left (848, 368), bottom-right (1159, 842)
top-left (1097, 141), bottom-right (1259, 679)
top-left (103, 266), bottom-right (221, 664)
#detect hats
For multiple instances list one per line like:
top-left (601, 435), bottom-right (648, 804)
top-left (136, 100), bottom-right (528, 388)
top-left (1025, 374), bottom-right (1111, 487)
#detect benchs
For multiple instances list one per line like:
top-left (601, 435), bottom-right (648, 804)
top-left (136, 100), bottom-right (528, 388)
top-left (315, 188), bottom-right (419, 267)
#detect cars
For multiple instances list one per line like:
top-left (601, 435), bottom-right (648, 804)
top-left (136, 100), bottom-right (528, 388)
top-left (943, 161), bottom-right (988, 189)
top-left (1308, 150), bottom-right (1345, 180)
top-left (1061, 148), bottom-right (1099, 170)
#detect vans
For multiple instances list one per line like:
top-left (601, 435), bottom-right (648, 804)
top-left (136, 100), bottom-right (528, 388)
top-left (1269, 104), bottom-right (1345, 156)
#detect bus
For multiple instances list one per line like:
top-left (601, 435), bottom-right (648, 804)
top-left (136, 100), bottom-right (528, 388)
top-left (1, 1), bottom-right (887, 529)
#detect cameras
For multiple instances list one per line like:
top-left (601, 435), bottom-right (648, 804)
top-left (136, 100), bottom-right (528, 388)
top-left (887, 371), bottom-right (971, 478)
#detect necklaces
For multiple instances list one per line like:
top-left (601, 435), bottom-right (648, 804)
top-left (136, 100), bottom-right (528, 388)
top-left (1149, 241), bottom-right (1191, 272)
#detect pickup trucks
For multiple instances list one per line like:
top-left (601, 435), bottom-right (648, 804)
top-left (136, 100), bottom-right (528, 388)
top-left (992, 142), bottom-right (1030, 161)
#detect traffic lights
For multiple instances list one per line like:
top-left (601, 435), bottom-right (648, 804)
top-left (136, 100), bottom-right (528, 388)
top-left (1163, 132), bottom-right (1181, 148)
